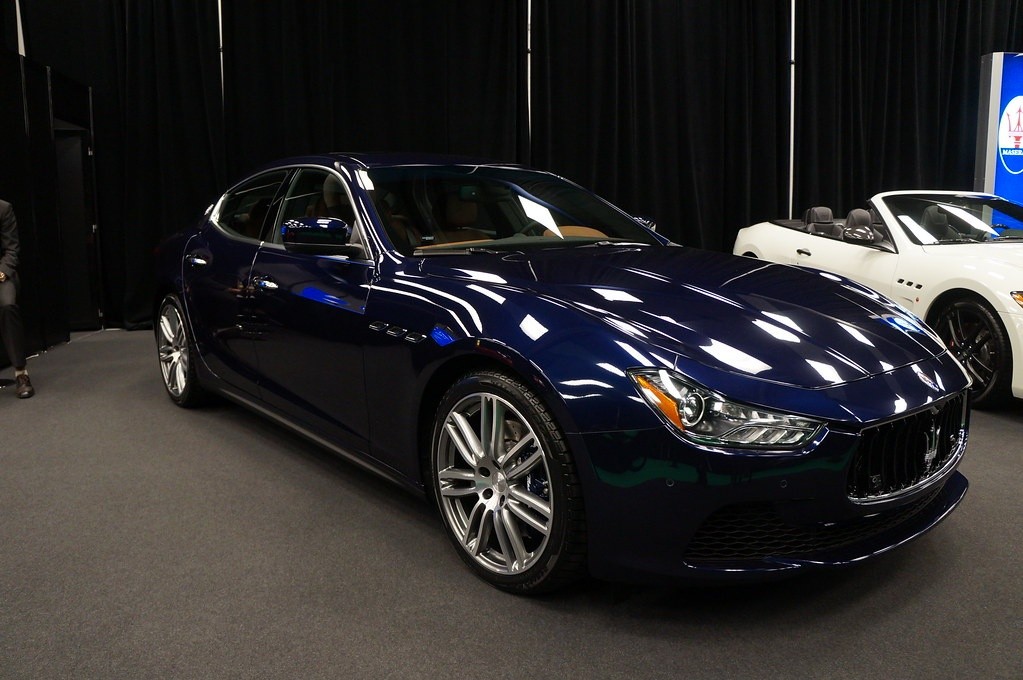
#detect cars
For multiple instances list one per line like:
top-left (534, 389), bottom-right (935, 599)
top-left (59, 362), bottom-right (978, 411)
top-left (149, 152), bottom-right (974, 600)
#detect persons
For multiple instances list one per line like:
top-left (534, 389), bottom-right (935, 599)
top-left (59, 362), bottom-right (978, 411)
top-left (0, 200), bottom-right (35, 399)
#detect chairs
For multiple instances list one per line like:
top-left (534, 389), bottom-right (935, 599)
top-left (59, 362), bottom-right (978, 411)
top-left (377, 200), bottom-right (420, 246)
top-left (803, 206), bottom-right (964, 244)
top-left (431, 199), bottom-right (488, 243)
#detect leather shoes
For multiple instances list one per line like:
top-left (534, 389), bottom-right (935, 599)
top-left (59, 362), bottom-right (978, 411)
top-left (16, 373), bottom-right (35, 397)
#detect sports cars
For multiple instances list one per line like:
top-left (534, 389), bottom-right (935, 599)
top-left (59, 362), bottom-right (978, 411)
top-left (731, 189), bottom-right (1023, 411)
